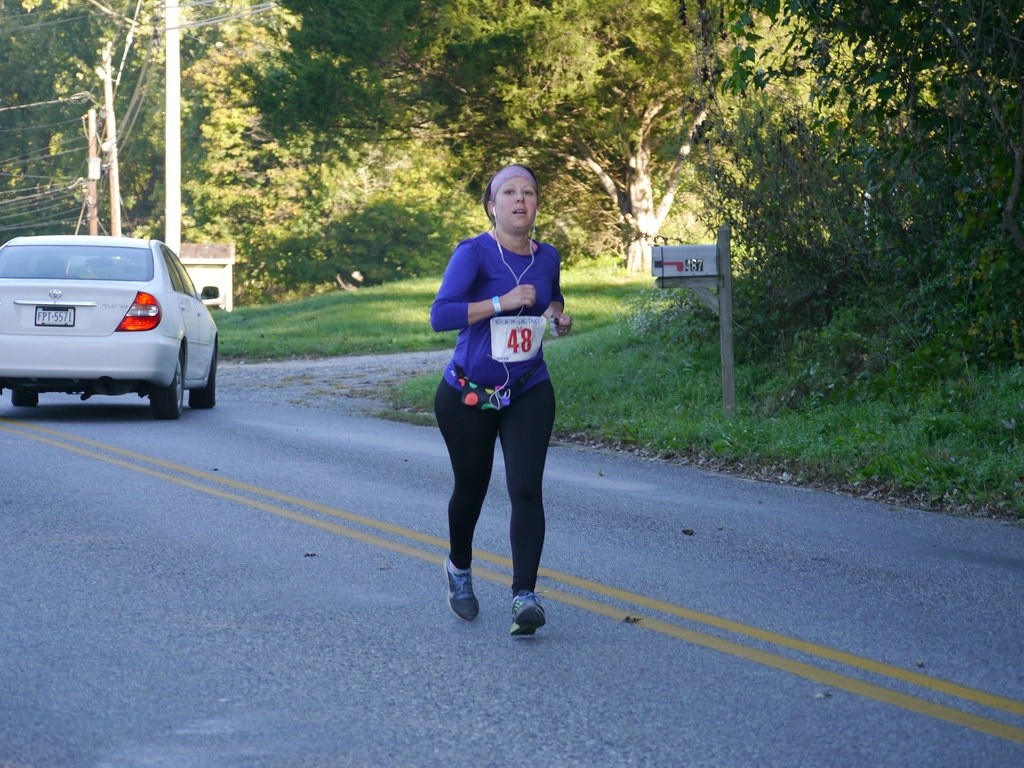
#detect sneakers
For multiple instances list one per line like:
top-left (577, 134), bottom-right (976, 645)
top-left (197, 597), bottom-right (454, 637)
top-left (444, 556), bottom-right (480, 623)
top-left (509, 590), bottom-right (550, 635)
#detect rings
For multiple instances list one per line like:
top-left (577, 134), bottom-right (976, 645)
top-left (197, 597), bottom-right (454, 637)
top-left (565, 329), bottom-right (568, 334)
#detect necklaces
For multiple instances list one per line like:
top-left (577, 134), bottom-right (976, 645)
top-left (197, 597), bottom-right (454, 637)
top-left (497, 237), bottom-right (529, 254)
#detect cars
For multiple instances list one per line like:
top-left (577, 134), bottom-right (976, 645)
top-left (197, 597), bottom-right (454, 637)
top-left (0, 234), bottom-right (219, 420)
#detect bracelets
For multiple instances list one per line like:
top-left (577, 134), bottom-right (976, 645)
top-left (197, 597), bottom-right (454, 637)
top-left (492, 295), bottom-right (503, 315)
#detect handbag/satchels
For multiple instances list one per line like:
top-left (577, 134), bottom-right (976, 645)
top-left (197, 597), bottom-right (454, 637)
top-left (449, 357), bottom-right (544, 409)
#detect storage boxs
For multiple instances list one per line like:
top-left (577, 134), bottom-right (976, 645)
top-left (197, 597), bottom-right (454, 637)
top-left (651, 245), bottom-right (720, 278)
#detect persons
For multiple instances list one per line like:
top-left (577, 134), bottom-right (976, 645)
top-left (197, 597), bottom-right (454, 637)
top-left (430, 163), bottom-right (573, 638)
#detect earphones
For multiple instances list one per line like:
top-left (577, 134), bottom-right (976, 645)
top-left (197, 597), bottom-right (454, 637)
top-left (492, 206), bottom-right (498, 218)
